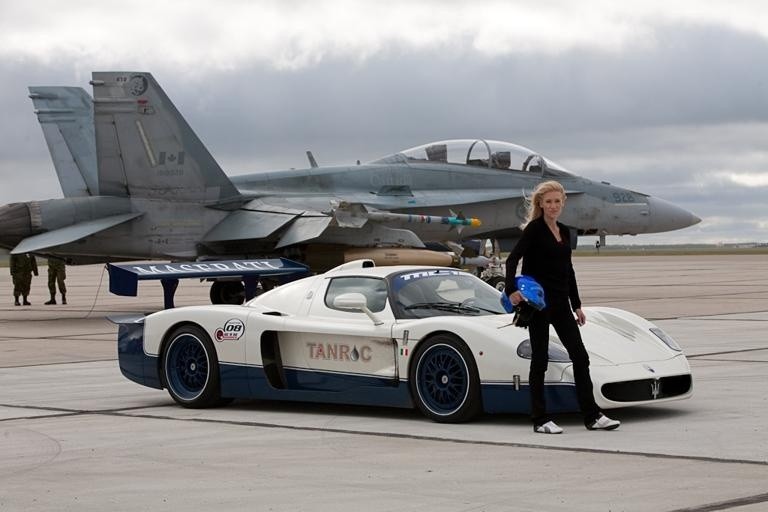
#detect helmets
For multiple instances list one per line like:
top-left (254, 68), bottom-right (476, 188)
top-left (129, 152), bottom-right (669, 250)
top-left (500, 275), bottom-right (546, 314)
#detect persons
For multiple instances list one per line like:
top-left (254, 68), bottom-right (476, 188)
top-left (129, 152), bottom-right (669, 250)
top-left (44, 260), bottom-right (66, 305)
top-left (10, 252), bottom-right (38, 306)
top-left (596, 241), bottom-right (600, 255)
top-left (505, 181), bottom-right (621, 433)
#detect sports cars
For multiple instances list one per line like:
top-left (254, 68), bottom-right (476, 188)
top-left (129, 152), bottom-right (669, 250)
top-left (105, 255), bottom-right (693, 423)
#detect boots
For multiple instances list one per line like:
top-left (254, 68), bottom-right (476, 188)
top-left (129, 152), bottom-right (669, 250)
top-left (14, 293), bottom-right (66, 306)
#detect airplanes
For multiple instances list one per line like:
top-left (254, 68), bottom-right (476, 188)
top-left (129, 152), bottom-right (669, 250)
top-left (0, 69), bottom-right (702, 306)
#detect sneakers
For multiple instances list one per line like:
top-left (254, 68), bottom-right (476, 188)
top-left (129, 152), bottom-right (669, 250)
top-left (536, 421), bottom-right (563, 434)
top-left (591, 415), bottom-right (620, 430)
